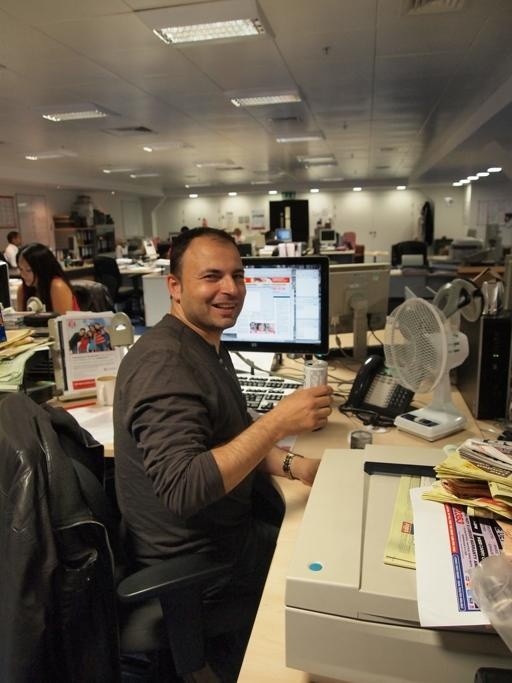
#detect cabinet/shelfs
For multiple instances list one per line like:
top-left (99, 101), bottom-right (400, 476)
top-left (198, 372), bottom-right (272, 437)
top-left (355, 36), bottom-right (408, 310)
top-left (55, 222), bottom-right (115, 270)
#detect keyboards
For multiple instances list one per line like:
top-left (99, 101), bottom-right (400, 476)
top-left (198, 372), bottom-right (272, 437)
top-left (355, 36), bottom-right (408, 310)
top-left (239, 375), bottom-right (303, 412)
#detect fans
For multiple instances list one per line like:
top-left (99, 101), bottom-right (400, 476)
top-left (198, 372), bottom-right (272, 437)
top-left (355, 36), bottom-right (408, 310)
top-left (381, 297), bottom-right (477, 440)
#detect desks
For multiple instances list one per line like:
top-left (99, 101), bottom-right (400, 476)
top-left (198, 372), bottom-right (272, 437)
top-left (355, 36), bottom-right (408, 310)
top-left (85, 355), bottom-right (509, 682)
top-left (62, 261), bottom-right (94, 280)
top-left (387, 264), bottom-right (508, 300)
top-left (321, 249), bottom-right (355, 264)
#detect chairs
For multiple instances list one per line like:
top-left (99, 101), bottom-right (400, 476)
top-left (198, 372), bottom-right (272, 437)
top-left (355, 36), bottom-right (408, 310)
top-left (66, 279), bottom-right (115, 312)
top-left (91, 255), bottom-right (147, 324)
top-left (2, 390), bottom-right (247, 681)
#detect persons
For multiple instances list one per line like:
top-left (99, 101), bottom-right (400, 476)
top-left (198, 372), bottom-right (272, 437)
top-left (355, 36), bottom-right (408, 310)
top-left (165, 226), bottom-right (189, 259)
top-left (16, 242), bottom-right (80, 315)
top-left (2, 230), bottom-right (22, 269)
top-left (232, 227), bottom-right (246, 242)
top-left (70, 321), bottom-right (113, 353)
top-left (110, 225), bottom-right (335, 607)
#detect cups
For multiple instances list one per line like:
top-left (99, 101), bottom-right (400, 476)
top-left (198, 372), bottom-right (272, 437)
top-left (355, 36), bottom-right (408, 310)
top-left (95, 376), bottom-right (116, 408)
top-left (350, 431), bottom-right (372, 449)
top-left (481, 280), bottom-right (499, 315)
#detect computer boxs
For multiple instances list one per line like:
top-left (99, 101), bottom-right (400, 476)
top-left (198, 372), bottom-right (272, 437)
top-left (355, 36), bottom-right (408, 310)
top-left (457, 310), bottom-right (512, 420)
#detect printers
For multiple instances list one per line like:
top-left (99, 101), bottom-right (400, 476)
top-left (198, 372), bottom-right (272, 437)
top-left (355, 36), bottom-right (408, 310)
top-left (285, 445), bottom-right (512, 683)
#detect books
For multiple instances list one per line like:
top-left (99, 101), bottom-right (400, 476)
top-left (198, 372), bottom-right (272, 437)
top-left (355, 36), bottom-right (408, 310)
top-left (55, 211), bottom-right (115, 260)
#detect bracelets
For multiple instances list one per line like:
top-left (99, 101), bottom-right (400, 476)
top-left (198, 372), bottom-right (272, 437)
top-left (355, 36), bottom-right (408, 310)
top-left (282, 450), bottom-right (304, 481)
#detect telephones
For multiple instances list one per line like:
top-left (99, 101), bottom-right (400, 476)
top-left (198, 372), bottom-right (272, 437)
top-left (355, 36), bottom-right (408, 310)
top-left (346, 355), bottom-right (414, 419)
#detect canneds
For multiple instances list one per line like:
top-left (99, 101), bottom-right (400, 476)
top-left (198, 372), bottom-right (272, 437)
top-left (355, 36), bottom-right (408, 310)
top-left (304, 360), bottom-right (328, 389)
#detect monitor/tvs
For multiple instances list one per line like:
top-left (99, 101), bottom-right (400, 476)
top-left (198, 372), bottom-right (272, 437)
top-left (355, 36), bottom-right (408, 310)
top-left (276, 228), bottom-right (292, 243)
top-left (142, 239), bottom-right (157, 258)
top-left (219, 254), bottom-right (331, 375)
top-left (319, 229), bottom-right (337, 244)
top-left (0, 262), bottom-right (11, 307)
top-left (330, 263), bottom-right (390, 365)
top-left (170, 234), bottom-right (178, 243)
